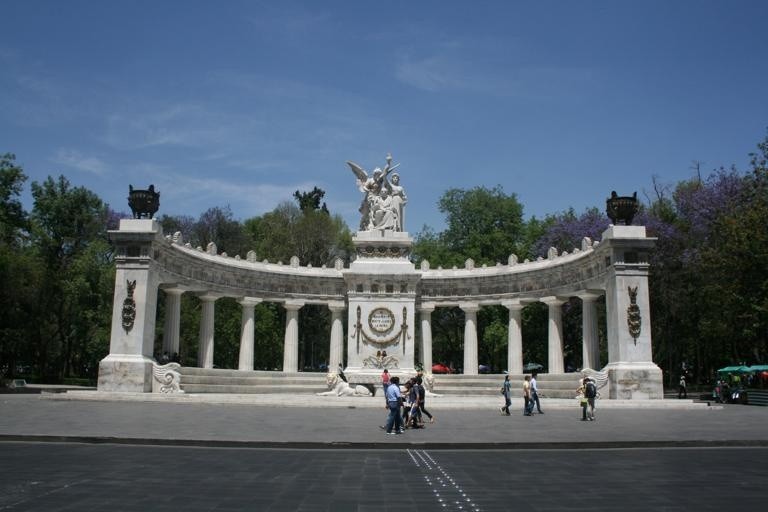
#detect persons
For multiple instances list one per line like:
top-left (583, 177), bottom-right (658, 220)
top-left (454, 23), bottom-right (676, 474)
top-left (526, 371), bottom-right (544, 414)
top-left (381, 161), bottom-right (408, 230)
top-left (382, 368), bottom-right (390, 395)
top-left (379, 375), bottom-right (434, 434)
top-left (522, 375), bottom-right (535, 416)
top-left (171, 352), bottom-right (178, 361)
top-left (712, 372), bottom-right (752, 404)
top-left (161, 351), bottom-right (169, 364)
top-left (581, 378), bottom-right (596, 419)
top-left (359, 167), bottom-right (382, 218)
top-left (575, 378), bottom-right (591, 420)
top-left (678, 376), bottom-right (687, 399)
top-left (337, 363), bottom-right (348, 383)
top-left (418, 362), bottom-right (424, 370)
top-left (499, 374), bottom-right (513, 415)
top-left (155, 352), bottom-right (160, 360)
top-left (371, 188), bottom-right (396, 226)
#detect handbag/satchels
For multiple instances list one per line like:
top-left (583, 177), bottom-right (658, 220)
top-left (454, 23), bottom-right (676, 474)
top-left (501, 388), bottom-right (504, 394)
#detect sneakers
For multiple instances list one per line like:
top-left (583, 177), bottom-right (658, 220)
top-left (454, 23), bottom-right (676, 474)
top-left (500, 409), bottom-right (510, 416)
top-left (379, 424), bottom-right (404, 435)
top-left (429, 416), bottom-right (434, 424)
